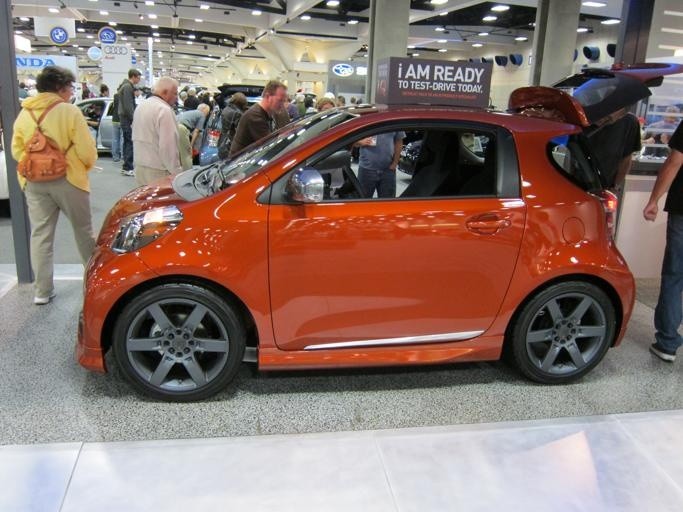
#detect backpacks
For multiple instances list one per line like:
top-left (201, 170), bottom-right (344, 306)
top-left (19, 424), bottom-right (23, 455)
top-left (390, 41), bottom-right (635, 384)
top-left (18, 99), bottom-right (73, 183)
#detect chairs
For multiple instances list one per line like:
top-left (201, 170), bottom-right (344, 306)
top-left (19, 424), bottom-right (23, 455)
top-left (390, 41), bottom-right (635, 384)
top-left (392, 143), bottom-right (456, 196)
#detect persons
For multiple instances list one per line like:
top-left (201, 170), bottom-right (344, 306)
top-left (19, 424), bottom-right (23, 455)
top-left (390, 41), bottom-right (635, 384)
top-left (639, 105), bottom-right (680, 155)
top-left (117, 67), bottom-right (141, 177)
top-left (642, 117), bottom-right (682, 362)
top-left (569, 106), bottom-right (643, 191)
top-left (8, 65), bottom-right (99, 304)
top-left (85, 75), bottom-right (408, 201)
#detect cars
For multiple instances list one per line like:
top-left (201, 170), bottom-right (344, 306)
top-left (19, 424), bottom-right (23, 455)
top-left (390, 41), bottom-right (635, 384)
top-left (198, 84), bottom-right (276, 166)
top-left (76, 63), bottom-right (683, 399)
top-left (72, 97), bottom-right (139, 156)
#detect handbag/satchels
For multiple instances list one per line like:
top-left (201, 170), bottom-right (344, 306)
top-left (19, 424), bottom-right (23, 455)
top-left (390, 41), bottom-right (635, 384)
top-left (219, 136), bottom-right (231, 155)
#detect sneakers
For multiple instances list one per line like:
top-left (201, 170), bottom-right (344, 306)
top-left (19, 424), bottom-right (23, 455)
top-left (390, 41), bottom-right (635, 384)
top-left (648, 342), bottom-right (676, 362)
top-left (121, 170), bottom-right (134, 176)
top-left (34, 290), bottom-right (56, 304)
